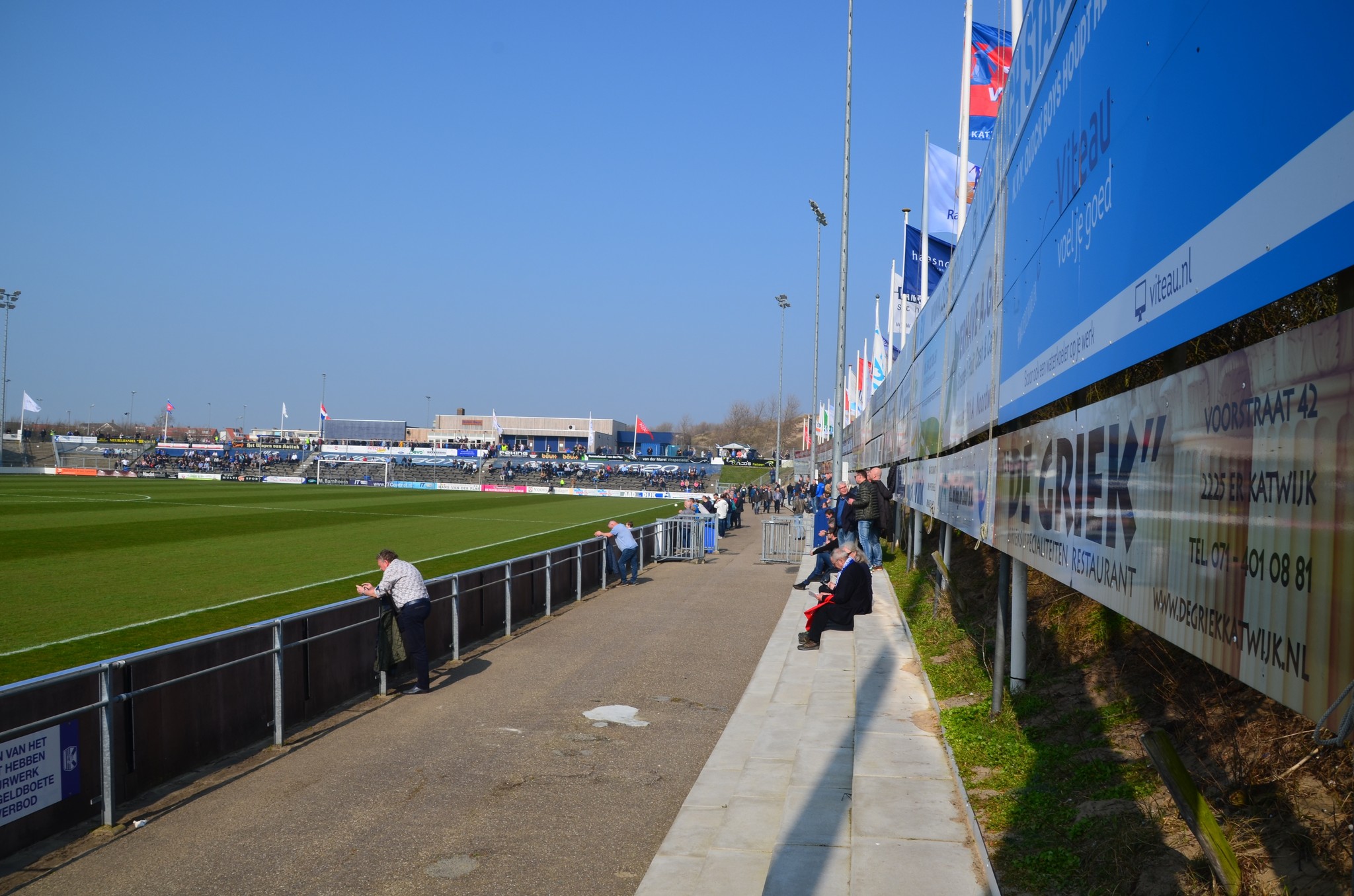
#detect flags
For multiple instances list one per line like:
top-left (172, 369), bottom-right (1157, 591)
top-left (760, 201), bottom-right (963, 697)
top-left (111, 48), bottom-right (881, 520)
top-left (589, 417), bottom-right (593, 446)
top-left (492, 412), bottom-right (503, 437)
top-left (320, 404), bottom-right (331, 420)
top-left (167, 400), bottom-right (175, 414)
top-left (636, 417), bottom-right (654, 440)
top-left (23, 392), bottom-right (41, 412)
top-left (803, 12), bottom-right (1012, 446)
top-left (283, 403), bottom-right (288, 418)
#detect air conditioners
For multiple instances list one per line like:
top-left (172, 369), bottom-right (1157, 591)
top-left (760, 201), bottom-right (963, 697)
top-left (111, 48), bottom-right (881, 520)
top-left (568, 425), bottom-right (575, 430)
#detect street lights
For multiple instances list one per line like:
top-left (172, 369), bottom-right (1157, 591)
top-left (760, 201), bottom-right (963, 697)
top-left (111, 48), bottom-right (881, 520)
top-left (66, 410), bottom-right (71, 432)
top-left (208, 403), bottom-right (211, 439)
top-left (322, 373), bottom-right (326, 404)
top-left (775, 294), bottom-right (791, 485)
top-left (425, 396), bottom-right (430, 428)
top-left (235, 416), bottom-right (243, 431)
top-left (129, 391), bottom-right (137, 436)
top-left (808, 198), bottom-right (829, 486)
top-left (87, 405), bottom-right (95, 436)
top-left (242, 405), bottom-right (246, 440)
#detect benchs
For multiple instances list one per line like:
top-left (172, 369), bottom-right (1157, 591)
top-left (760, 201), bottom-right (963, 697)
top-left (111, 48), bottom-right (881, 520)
top-left (299, 463), bottom-right (475, 484)
top-left (165, 456), bottom-right (300, 476)
top-left (484, 468), bottom-right (710, 493)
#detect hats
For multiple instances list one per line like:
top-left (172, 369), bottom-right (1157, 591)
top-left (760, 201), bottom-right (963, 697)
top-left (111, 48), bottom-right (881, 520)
top-left (713, 493), bottom-right (718, 496)
top-left (822, 475), bottom-right (825, 478)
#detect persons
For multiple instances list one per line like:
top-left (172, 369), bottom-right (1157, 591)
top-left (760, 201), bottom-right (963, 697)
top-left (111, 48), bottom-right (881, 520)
top-left (679, 467), bottom-right (894, 650)
top-left (50, 428), bottom-right (56, 442)
top-left (67, 428), bottom-right (299, 473)
top-left (306, 435), bottom-right (509, 475)
top-left (23, 428), bottom-right (31, 442)
top-left (6, 428), bottom-right (11, 441)
top-left (594, 520), bottom-right (639, 586)
top-left (721, 448), bottom-right (790, 460)
top-left (626, 521), bottom-right (633, 529)
top-left (40, 428), bottom-right (46, 442)
top-left (356, 549), bottom-right (431, 694)
top-left (481, 442), bottom-right (712, 495)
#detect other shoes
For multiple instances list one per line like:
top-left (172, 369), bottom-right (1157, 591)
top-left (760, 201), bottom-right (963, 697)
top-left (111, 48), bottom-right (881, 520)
top-left (807, 575), bottom-right (823, 582)
top-left (726, 525), bottom-right (741, 530)
top-left (869, 565), bottom-right (874, 573)
top-left (793, 582), bottom-right (805, 590)
top-left (718, 536), bottom-right (725, 539)
top-left (802, 537), bottom-right (804, 540)
top-left (752, 508), bottom-right (780, 514)
top-left (795, 538), bottom-right (800, 540)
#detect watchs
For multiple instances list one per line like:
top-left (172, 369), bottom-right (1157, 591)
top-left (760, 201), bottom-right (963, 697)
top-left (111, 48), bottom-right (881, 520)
top-left (362, 590), bottom-right (365, 595)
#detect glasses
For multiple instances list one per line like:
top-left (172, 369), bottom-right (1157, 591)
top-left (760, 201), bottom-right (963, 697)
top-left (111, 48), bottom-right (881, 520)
top-left (827, 533), bottom-right (832, 535)
top-left (854, 475), bottom-right (861, 479)
top-left (847, 552), bottom-right (853, 556)
top-left (379, 552), bottom-right (387, 558)
top-left (839, 486), bottom-right (847, 490)
top-left (832, 559), bottom-right (839, 567)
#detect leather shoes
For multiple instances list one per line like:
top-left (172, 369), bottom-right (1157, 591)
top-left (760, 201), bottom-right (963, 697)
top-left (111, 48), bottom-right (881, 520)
top-left (402, 685), bottom-right (429, 694)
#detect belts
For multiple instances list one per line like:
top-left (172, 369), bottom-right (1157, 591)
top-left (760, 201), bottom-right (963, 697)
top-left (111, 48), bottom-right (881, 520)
top-left (403, 598), bottom-right (431, 607)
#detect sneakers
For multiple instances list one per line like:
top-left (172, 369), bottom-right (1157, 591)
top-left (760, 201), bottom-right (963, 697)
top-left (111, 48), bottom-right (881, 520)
top-left (874, 565), bottom-right (883, 570)
top-left (798, 632), bottom-right (808, 639)
top-left (615, 582), bottom-right (628, 587)
top-left (797, 640), bottom-right (820, 650)
top-left (628, 582), bottom-right (636, 586)
top-left (799, 637), bottom-right (810, 644)
